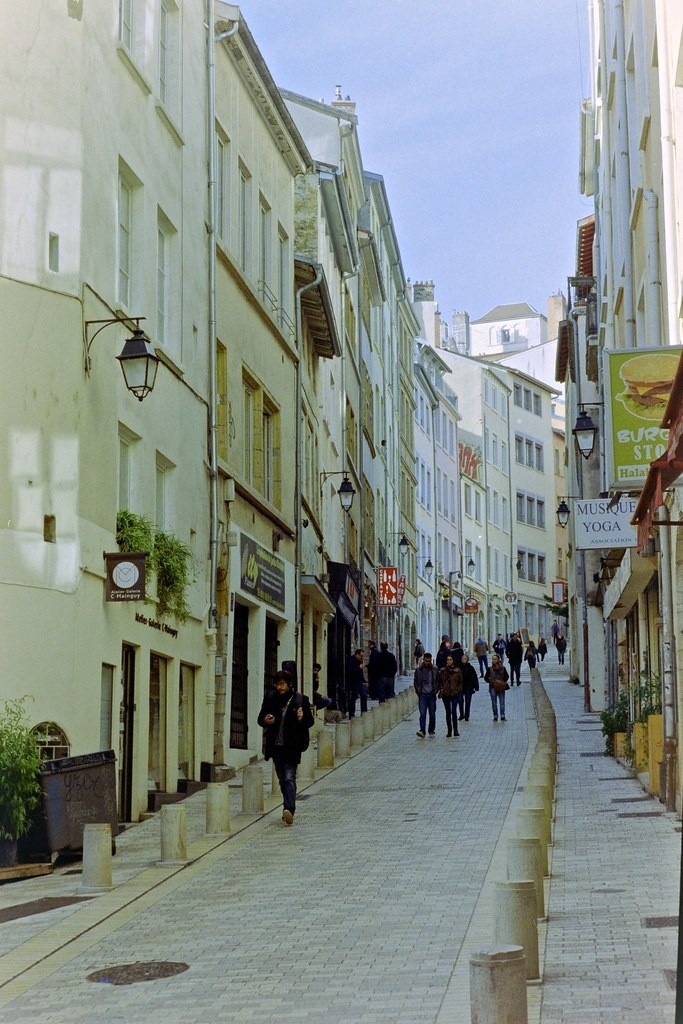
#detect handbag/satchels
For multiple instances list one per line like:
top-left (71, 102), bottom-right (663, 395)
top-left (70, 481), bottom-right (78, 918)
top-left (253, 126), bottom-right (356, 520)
top-left (494, 679), bottom-right (506, 691)
top-left (526, 649), bottom-right (532, 658)
top-left (438, 689), bottom-right (444, 698)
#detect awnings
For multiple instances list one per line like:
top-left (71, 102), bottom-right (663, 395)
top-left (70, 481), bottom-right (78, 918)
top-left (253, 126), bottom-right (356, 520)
top-left (629, 349), bottom-right (683, 556)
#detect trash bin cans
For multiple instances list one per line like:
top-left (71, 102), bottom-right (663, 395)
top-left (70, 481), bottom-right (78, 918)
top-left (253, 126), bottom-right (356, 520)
top-left (40, 748), bottom-right (120, 866)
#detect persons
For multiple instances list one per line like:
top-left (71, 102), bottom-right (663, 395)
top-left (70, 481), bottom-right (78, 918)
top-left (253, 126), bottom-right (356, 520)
top-left (538, 638), bottom-right (548, 661)
top-left (437, 635), bottom-right (464, 669)
top-left (458, 654), bottom-right (479, 721)
top-left (505, 634), bottom-right (523, 686)
top-left (493, 633), bottom-right (506, 665)
top-left (553, 629), bottom-right (566, 665)
top-left (376, 641), bottom-right (398, 705)
top-left (439, 655), bottom-right (463, 737)
top-left (367, 640), bottom-right (380, 701)
top-left (474, 637), bottom-right (489, 677)
top-left (484, 654), bottom-right (509, 722)
top-left (414, 639), bottom-right (421, 666)
top-left (414, 654), bottom-right (440, 738)
top-left (524, 641), bottom-right (539, 670)
top-left (347, 649), bottom-right (369, 719)
top-left (550, 620), bottom-right (559, 645)
top-left (313, 661), bottom-right (331, 715)
top-left (257, 671), bottom-right (315, 824)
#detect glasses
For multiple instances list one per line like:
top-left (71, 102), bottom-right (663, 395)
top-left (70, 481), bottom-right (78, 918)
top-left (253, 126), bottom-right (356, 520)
top-left (274, 681), bottom-right (285, 686)
top-left (358, 654), bottom-right (363, 657)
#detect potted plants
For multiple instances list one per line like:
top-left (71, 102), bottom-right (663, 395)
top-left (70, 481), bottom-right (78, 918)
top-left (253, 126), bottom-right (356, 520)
top-left (600, 667), bottom-right (665, 800)
top-left (0, 694), bottom-right (58, 863)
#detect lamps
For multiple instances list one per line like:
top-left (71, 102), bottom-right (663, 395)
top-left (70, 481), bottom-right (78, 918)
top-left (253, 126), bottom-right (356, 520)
top-left (389, 531), bottom-right (411, 558)
top-left (510, 557), bottom-right (523, 572)
top-left (416, 555), bottom-right (434, 577)
top-left (318, 469), bottom-right (356, 513)
top-left (463, 553), bottom-right (477, 574)
top-left (558, 494), bottom-right (581, 530)
top-left (570, 401), bottom-right (604, 460)
top-left (82, 312), bottom-right (162, 402)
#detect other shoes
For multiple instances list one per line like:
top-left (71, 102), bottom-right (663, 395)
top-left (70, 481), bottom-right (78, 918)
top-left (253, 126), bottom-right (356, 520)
top-left (458, 716), bottom-right (465, 720)
top-left (501, 717), bottom-right (507, 721)
top-left (416, 731), bottom-right (425, 738)
top-left (429, 732), bottom-right (435, 735)
top-left (465, 718), bottom-right (469, 721)
top-left (493, 718), bottom-right (498, 721)
top-left (446, 733), bottom-right (452, 737)
top-left (454, 733), bottom-right (460, 736)
top-left (281, 809), bottom-right (293, 824)
